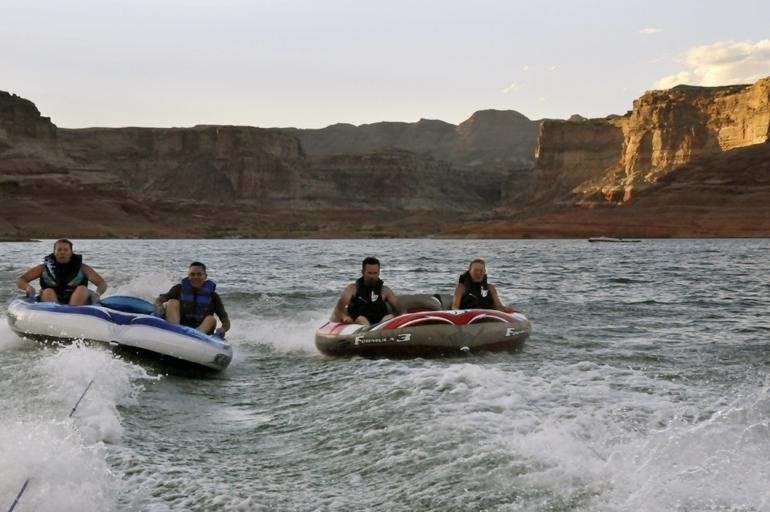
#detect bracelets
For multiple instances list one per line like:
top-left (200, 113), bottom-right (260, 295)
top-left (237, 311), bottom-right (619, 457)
top-left (95, 290), bottom-right (104, 300)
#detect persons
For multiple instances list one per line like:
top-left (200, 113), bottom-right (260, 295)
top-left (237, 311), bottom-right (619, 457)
top-left (17, 238), bottom-right (108, 306)
top-left (334, 256), bottom-right (406, 325)
top-left (452, 258), bottom-right (515, 314)
top-left (157, 261), bottom-right (230, 336)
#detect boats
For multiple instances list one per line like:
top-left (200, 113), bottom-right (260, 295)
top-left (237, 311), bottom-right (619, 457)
top-left (5, 288), bottom-right (234, 376)
top-left (314, 293), bottom-right (531, 360)
